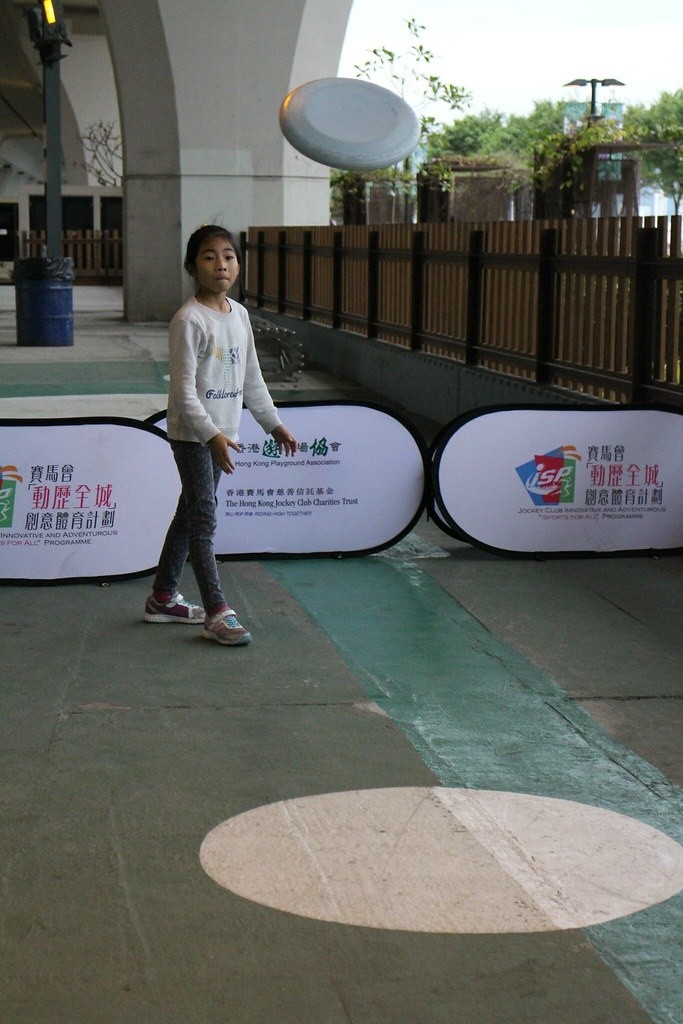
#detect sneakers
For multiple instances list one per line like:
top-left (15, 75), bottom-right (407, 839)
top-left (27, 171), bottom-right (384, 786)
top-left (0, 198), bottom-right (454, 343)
top-left (144, 588), bottom-right (206, 624)
top-left (201, 603), bottom-right (253, 645)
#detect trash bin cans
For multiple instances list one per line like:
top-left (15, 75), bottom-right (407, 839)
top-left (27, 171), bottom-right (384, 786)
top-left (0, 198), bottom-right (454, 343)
top-left (11, 255), bottom-right (75, 348)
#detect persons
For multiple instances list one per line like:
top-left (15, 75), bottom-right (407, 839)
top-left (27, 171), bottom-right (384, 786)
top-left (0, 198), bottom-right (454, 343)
top-left (143, 224), bottom-right (297, 645)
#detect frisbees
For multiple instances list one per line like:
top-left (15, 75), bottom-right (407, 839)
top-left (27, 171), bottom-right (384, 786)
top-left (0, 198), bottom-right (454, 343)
top-left (278, 77), bottom-right (421, 171)
